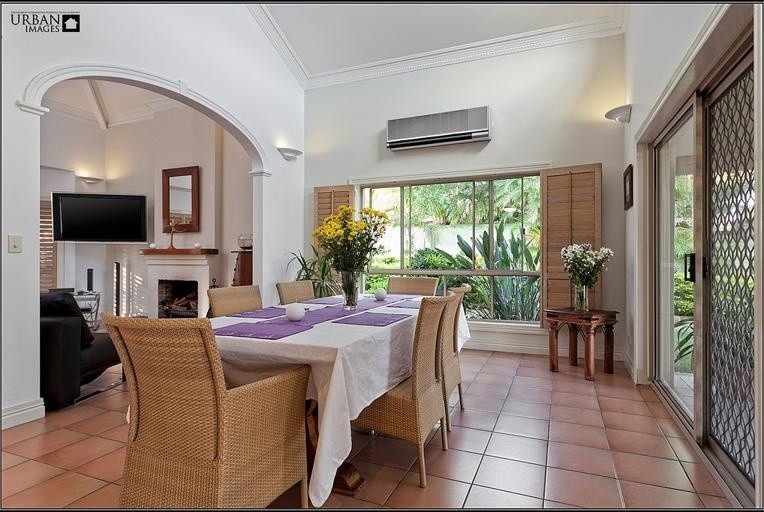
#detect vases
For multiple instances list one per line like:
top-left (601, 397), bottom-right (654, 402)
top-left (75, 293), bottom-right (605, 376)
top-left (342, 271), bottom-right (359, 310)
top-left (574, 284), bottom-right (589, 313)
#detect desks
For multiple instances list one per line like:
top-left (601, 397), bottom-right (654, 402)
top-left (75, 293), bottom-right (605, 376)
top-left (61, 292), bottom-right (101, 331)
top-left (545, 308), bottom-right (618, 380)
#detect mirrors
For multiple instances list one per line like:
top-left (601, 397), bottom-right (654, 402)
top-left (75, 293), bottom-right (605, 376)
top-left (160, 166), bottom-right (201, 234)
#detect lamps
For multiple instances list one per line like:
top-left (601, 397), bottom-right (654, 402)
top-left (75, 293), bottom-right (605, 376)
top-left (78, 175), bottom-right (103, 187)
top-left (604, 104), bottom-right (631, 123)
top-left (277, 142), bottom-right (303, 163)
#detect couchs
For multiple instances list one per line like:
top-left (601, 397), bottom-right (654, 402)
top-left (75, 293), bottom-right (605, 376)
top-left (39, 292), bottom-right (127, 409)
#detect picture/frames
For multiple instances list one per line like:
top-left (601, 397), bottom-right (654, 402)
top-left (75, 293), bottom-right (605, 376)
top-left (622, 165), bottom-right (635, 210)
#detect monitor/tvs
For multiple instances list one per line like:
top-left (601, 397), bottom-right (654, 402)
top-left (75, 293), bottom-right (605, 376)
top-left (51, 191), bottom-right (148, 246)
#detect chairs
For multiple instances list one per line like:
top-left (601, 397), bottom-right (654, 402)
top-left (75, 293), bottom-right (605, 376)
top-left (387, 276), bottom-right (439, 296)
top-left (100, 314), bottom-right (314, 510)
top-left (436, 282), bottom-right (472, 432)
top-left (349, 291), bottom-right (449, 487)
top-left (276, 280), bottom-right (315, 305)
top-left (207, 283), bottom-right (263, 317)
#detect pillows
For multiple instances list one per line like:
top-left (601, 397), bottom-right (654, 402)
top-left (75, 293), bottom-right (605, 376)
top-left (40, 291), bottom-right (94, 351)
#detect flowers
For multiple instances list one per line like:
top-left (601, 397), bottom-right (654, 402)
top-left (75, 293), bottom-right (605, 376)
top-left (311, 205), bottom-right (390, 308)
top-left (559, 244), bottom-right (612, 312)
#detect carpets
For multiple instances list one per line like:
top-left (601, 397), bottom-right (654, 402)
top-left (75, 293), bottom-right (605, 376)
top-left (75, 334), bottom-right (125, 407)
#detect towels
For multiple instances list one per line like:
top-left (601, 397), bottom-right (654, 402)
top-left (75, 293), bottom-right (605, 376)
top-left (212, 322), bottom-right (313, 340)
top-left (363, 290), bottom-right (424, 301)
top-left (298, 295), bottom-right (344, 304)
top-left (227, 306), bottom-right (308, 319)
top-left (388, 301), bottom-right (425, 309)
top-left (332, 312), bottom-right (412, 327)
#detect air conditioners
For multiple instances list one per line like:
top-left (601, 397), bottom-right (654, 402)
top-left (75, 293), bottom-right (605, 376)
top-left (385, 105), bottom-right (492, 152)
